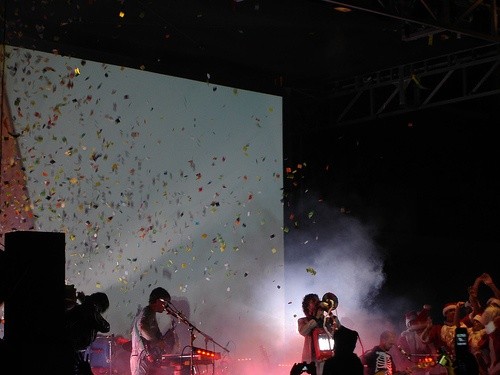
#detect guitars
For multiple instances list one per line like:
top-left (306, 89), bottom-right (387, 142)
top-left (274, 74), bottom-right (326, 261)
top-left (147, 323), bottom-right (176, 364)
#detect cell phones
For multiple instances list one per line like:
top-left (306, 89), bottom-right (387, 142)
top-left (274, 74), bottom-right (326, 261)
top-left (454, 327), bottom-right (468, 375)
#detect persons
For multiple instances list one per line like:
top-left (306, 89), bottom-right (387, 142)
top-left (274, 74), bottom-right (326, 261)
top-left (322, 326), bottom-right (364, 375)
top-left (64, 285), bottom-right (109, 375)
top-left (163, 298), bottom-right (192, 353)
top-left (360, 331), bottom-right (412, 375)
top-left (397, 274), bottom-right (500, 375)
top-left (290, 361), bottom-right (317, 375)
top-left (298, 294), bottom-right (340, 375)
top-left (129, 287), bottom-right (171, 375)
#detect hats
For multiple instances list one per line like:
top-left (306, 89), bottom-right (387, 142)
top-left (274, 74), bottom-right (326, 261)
top-left (443, 302), bottom-right (457, 316)
top-left (473, 312), bottom-right (484, 325)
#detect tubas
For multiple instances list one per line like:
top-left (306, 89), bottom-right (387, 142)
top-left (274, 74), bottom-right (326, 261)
top-left (316, 292), bottom-right (340, 334)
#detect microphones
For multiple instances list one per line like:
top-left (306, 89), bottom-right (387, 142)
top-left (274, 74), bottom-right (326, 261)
top-left (398, 345), bottom-right (410, 360)
top-left (160, 297), bottom-right (170, 304)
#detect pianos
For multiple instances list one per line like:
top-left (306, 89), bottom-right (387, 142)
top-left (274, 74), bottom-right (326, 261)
top-left (162, 347), bottom-right (221, 365)
